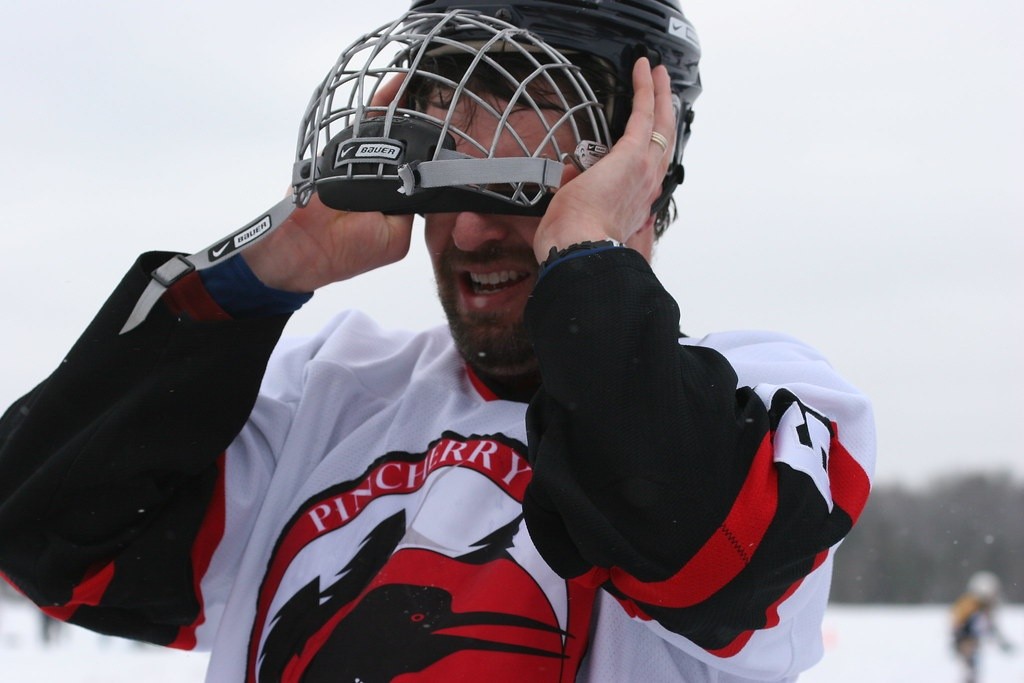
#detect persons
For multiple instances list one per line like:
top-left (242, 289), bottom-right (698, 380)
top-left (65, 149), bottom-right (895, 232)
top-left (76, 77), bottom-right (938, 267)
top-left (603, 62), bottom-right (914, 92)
top-left (0, 0), bottom-right (879, 683)
top-left (941, 569), bottom-right (1013, 683)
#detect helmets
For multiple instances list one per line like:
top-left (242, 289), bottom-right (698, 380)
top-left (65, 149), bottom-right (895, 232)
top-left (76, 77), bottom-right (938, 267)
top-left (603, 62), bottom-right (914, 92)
top-left (290, 0), bottom-right (700, 218)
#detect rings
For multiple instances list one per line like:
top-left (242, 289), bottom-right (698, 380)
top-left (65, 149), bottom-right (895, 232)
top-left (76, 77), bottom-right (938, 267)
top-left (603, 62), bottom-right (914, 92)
top-left (651, 131), bottom-right (668, 152)
